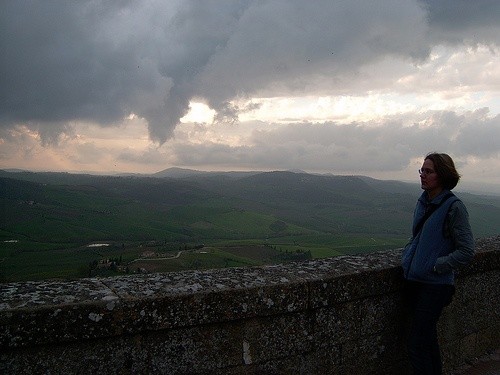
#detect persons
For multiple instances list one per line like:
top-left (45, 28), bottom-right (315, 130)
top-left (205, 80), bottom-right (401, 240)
top-left (400, 152), bottom-right (476, 375)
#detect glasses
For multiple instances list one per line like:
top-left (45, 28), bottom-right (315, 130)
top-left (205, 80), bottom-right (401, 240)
top-left (418, 168), bottom-right (437, 175)
